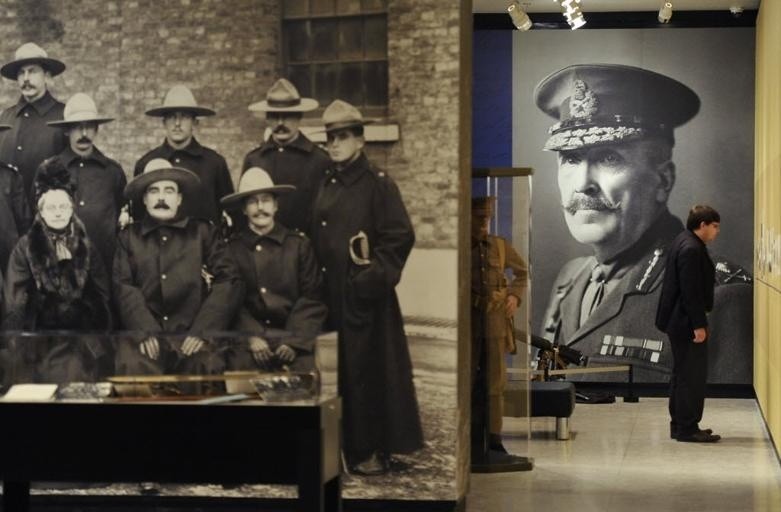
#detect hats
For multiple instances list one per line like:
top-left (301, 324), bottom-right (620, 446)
top-left (246, 76), bottom-right (321, 114)
top-left (121, 157), bottom-right (204, 205)
top-left (0, 41), bottom-right (67, 81)
top-left (308, 98), bottom-right (376, 136)
top-left (533, 59), bottom-right (703, 153)
top-left (0, 124), bottom-right (13, 133)
top-left (143, 83), bottom-right (217, 118)
top-left (45, 92), bottom-right (116, 128)
top-left (470, 195), bottom-right (499, 218)
top-left (32, 155), bottom-right (79, 204)
top-left (219, 165), bottom-right (298, 213)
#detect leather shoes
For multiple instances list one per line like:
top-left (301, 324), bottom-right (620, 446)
top-left (675, 426), bottom-right (722, 443)
top-left (488, 440), bottom-right (514, 463)
top-left (351, 448), bottom-right (393, 477)
top-left (669, 420), bottom-right (713, 440)
top-left (137, 480), bottom-right (161, 495)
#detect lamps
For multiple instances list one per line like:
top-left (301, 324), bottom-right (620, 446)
top-left (656, 1), bottom-right (675, 25)
top-left (504, 0), bottom-right (537, 34)
top-left (552, 0), bottom-right (588, 33)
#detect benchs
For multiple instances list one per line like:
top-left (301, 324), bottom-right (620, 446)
top-left (499, 377), bottom-right (577, 443)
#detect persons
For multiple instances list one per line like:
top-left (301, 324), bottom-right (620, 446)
top-left (3, 185), bottom-right (120, 383)
top-left (28, 90), bottom-right (127, 331)
top-left (0, 40), bottom-right (69, 275)
top-left (219, 163), bottom-right (327, 492)
top-left (653, 203), bottom-right (722, 444)
top-left (108, 157), bottom-right (248, 496)
top-left (240, 75), bottom-right (331, 240)
top-left (529, 62), bottom-right (722, 385)
top-left (471, 193), bottom-right (532, 461)
top-left (305, 98), bottom-right (427, 474)
top-left (130, 80), bottom-right (233, 206)
